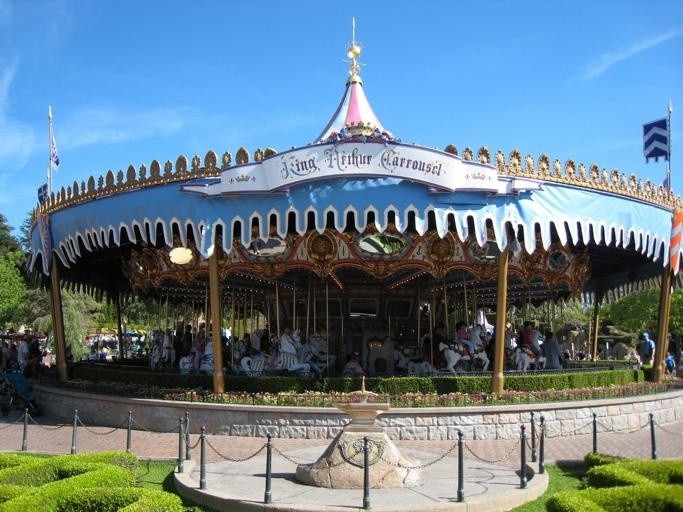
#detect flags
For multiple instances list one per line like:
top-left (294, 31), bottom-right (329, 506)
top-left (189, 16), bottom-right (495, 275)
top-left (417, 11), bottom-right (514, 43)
top-left (48, 123), bottom-right (60, 171)
top-left (642, 117), bottom-right (666, 164)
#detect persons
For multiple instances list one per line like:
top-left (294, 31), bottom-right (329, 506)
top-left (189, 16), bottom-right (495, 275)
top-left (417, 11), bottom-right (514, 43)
top-left (0, 318), bottom-right (47, 424)
top-left (665, 351), bottom-right (676, 374)
top-left (635, 332), bottom-right (655, 365)
